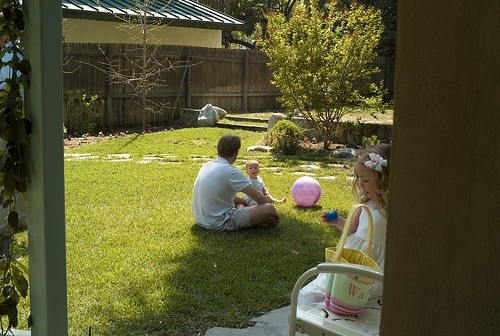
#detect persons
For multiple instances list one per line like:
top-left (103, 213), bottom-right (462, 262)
top-left (297, 144), bottom-right (391, 305)
top-left (191, 135), bottom-right (286, 231)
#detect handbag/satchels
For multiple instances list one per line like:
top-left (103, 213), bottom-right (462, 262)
top-left (325, 203), bottom-right (379, 316)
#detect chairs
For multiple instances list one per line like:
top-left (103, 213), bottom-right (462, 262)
top-left (289, 263), bottom-right (384, 336)
